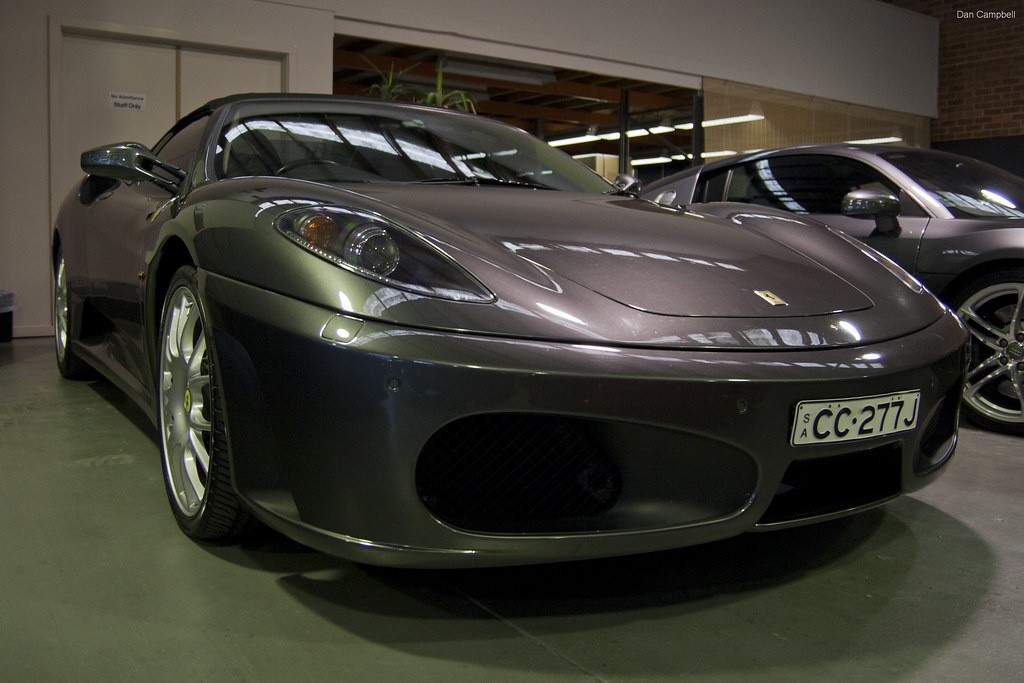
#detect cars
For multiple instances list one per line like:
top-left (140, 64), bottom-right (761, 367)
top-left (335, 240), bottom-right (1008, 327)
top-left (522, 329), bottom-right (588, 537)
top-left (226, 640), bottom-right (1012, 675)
top-left (637, 145), bottom-right (1024, 436)
top-left (50, 93), bottom-right (974, 574)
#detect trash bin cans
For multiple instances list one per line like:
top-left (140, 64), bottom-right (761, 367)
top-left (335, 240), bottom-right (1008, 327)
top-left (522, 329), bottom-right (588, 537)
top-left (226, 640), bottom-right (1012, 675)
top-left (0, 289), bottom-right (15, 343)
